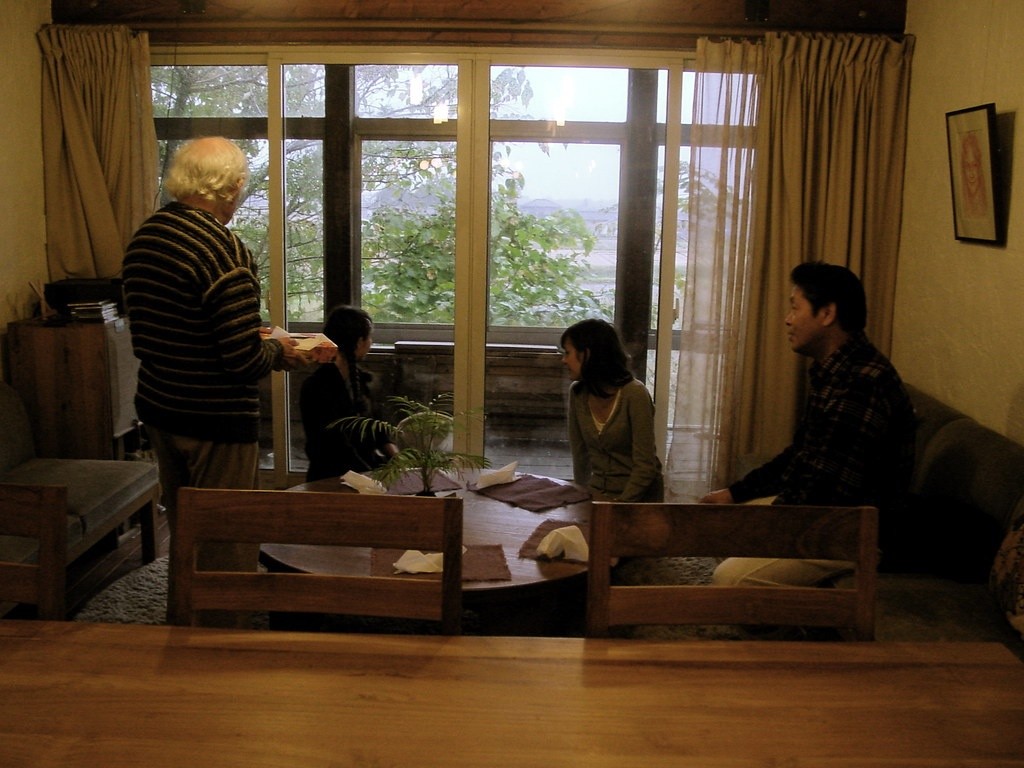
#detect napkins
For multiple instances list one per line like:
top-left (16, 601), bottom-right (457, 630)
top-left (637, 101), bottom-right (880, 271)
top-left (391, 546), bottom-right (467, 574)
top-left (339, 470), bottom-right (387, 495)
top-left (476, 460), bottom-right (522, 490)
top-left (537, 524), bottom-right (590, 562)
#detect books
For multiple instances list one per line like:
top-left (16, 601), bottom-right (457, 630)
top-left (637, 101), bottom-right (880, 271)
top-left (67, 299), bottom-right (119, 323)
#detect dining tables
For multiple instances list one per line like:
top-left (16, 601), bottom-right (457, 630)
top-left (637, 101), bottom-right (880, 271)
top-left (256, 468), bottom-right (596, 607)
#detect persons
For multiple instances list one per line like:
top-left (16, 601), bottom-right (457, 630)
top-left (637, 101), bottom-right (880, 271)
top-left (560, 319), bottom-right (663, 502)
top-left (122, 136), bottom-right (308, 628)
top-left (700, 262), bottom-right (915, 587)
top-left (300, 305), bottom-right (401, 482)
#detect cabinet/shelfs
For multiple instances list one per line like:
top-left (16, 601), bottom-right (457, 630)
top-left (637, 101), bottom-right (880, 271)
top-left (6, 310), bottom-right (170, 562)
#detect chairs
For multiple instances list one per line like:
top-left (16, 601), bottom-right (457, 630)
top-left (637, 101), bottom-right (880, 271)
top-left (0, 481), bottom-right (68, 623)
top-left (167, 486), bottom-right (463, 636)
top-left (585, 501), bottom-right (877, 641)
top-left (0, 381), bottom-right (159, 582)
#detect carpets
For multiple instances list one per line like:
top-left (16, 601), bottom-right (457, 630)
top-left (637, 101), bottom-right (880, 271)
top-left (73, 554), bottom-right (168, 624)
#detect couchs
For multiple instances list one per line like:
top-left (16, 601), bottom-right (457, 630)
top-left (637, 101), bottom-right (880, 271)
top-left (826, 380), bottom-right (1024, 645)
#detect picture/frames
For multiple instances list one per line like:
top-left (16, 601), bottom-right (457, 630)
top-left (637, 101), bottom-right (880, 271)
top-left (945, 103), bottom-right (1008, 247)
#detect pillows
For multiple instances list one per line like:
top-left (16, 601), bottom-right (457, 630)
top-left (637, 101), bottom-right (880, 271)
top-left (988, 496), bottom-right (1024, 642)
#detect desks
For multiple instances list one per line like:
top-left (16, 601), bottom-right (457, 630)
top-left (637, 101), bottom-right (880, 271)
top-left (0, 622), bottom-right (1024, 768)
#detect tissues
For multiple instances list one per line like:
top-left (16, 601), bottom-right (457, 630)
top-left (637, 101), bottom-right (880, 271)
top-left (258, 325), bottom-right (341, 362)
